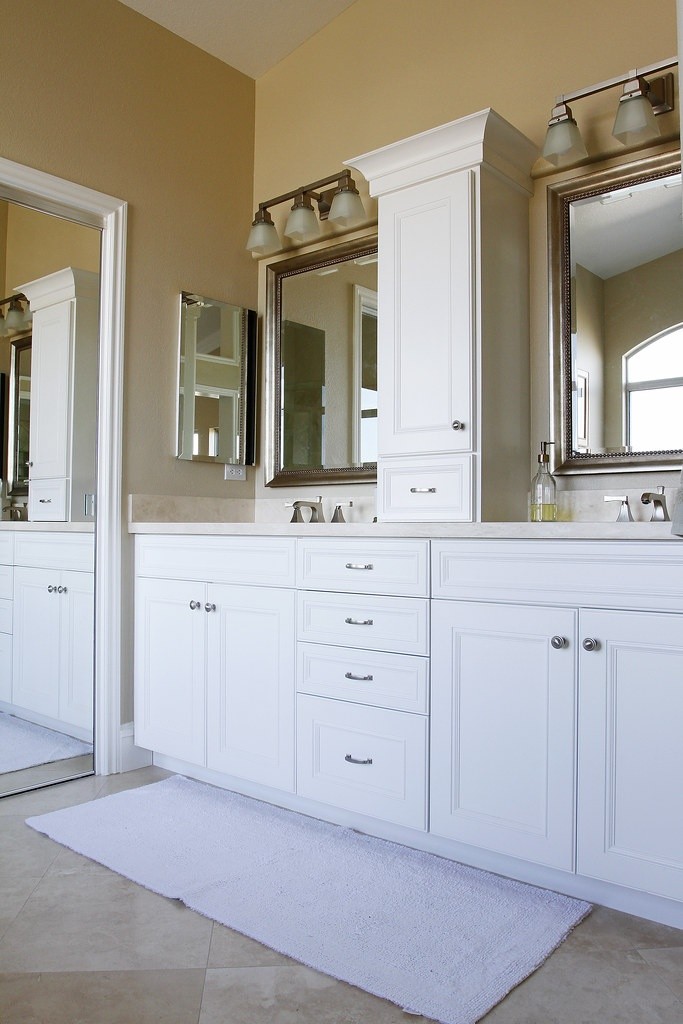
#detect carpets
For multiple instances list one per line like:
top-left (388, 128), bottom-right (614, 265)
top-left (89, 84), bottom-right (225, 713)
top-left (0, 711), bottom-right (93, 775)
top-left (24, 774), bottom-right (593, 1024)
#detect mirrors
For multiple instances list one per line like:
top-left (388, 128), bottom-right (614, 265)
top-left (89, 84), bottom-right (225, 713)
top-left (546, 146), bottom-right (683, 476)
top-left (265, 232), bottom-right (379, 487)
top-left (175, 290), bottom-right (247, 464)
top-left (6, 335), bottom-right (32, 496)
top-left (0, 184), bottom-right (96, 801)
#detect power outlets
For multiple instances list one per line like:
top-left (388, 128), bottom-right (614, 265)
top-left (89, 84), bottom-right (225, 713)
top-left (224, 464), bottom-right (246, 480)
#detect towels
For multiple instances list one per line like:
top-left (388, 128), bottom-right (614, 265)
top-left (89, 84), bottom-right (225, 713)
top-left (672, 464), bottom-right (683, 538)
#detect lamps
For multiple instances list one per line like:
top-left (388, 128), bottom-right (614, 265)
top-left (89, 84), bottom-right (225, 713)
top-left (0, 293), bottom-right (34, 336)
top-left (542, 56), bottom-right (679, 168)
top-left (244, 169), bottom-right (367, 256)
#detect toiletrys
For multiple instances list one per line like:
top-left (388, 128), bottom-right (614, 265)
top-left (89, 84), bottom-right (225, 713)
top-left (530, 442), bottom-right (558, 522)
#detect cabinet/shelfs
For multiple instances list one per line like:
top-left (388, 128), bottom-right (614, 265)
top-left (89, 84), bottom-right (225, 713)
top-left (376, 167), bottom-right (529, 522)
top-left (133, 533), bottom-right (682, 929)
top-left (30, 299), bottom-right (99, 523)
top-left (0, 530), bottom-right (94, 744)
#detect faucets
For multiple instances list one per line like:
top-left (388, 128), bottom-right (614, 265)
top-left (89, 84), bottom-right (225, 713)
top-left (283, 496), bottom-right (352, 523)
top-left (1, 502), bottom-right (28, 521)
top-left (641, 485), bottom-right (670, 521)
